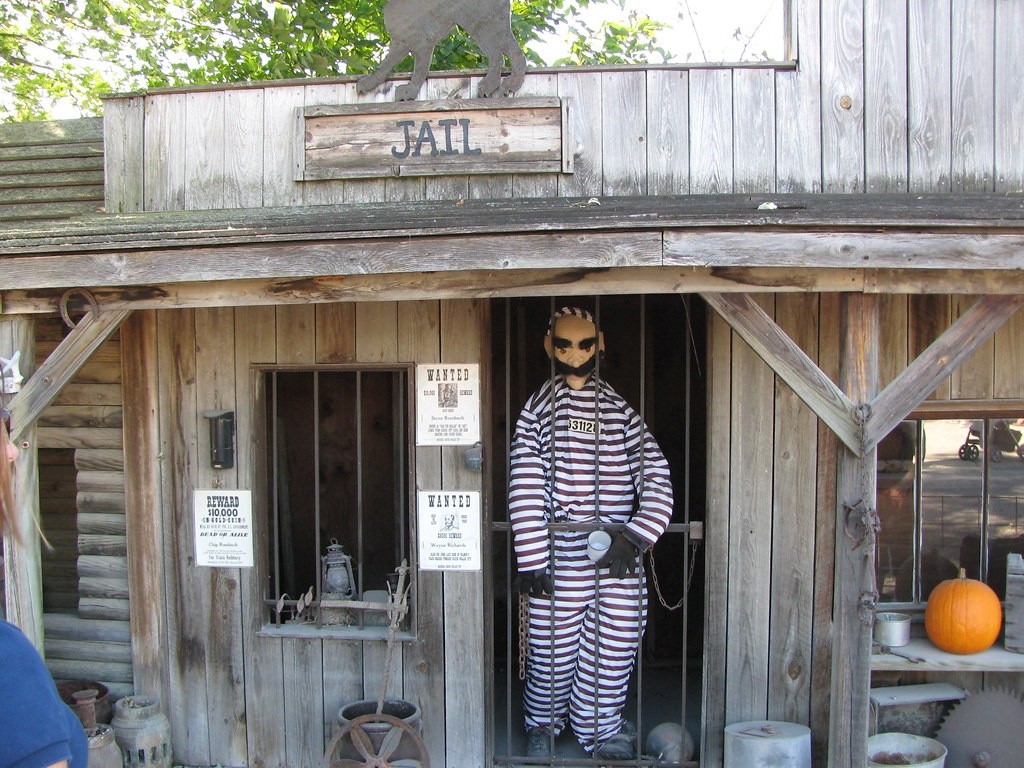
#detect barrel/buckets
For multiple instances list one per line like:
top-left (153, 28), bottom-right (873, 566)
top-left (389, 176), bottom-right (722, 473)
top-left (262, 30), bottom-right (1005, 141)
top-left (54, 679), bottom-right (112, 729)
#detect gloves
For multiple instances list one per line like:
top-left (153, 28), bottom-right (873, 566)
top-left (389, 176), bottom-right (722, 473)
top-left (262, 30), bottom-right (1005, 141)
top-left (512, 564), bottom-right (559, 598)
top-left (594, 525), bottom-right (638, 580)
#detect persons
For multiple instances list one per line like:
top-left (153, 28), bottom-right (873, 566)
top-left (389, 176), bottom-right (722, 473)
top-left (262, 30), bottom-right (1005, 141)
top-left (0, 621), bottom-right (88, 768)
top-left (876, 420), bottom-right (926, 601)
top-left (514, 308), bottom-right (673, 758)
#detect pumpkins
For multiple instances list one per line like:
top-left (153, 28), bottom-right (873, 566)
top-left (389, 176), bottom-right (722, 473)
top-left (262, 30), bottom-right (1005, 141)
top-left (924, 568), bottom-right (1002, 654)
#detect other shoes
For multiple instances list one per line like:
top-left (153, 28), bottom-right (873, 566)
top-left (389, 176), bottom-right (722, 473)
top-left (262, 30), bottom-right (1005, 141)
top-left (526, 732), bottom-right (550, 758)
top-left (591, 734), bottom-right (634, 760)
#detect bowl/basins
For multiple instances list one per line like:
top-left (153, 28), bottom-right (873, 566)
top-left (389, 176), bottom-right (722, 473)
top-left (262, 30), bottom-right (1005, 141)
top-left (873, 610), bottom-right (912, 647)
top-left (868, 731), bottom-right (948, 768)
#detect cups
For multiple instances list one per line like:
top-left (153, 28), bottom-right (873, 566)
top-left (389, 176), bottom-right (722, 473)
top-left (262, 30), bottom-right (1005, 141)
top-left (586, 526), bottom-right (612, 562)
